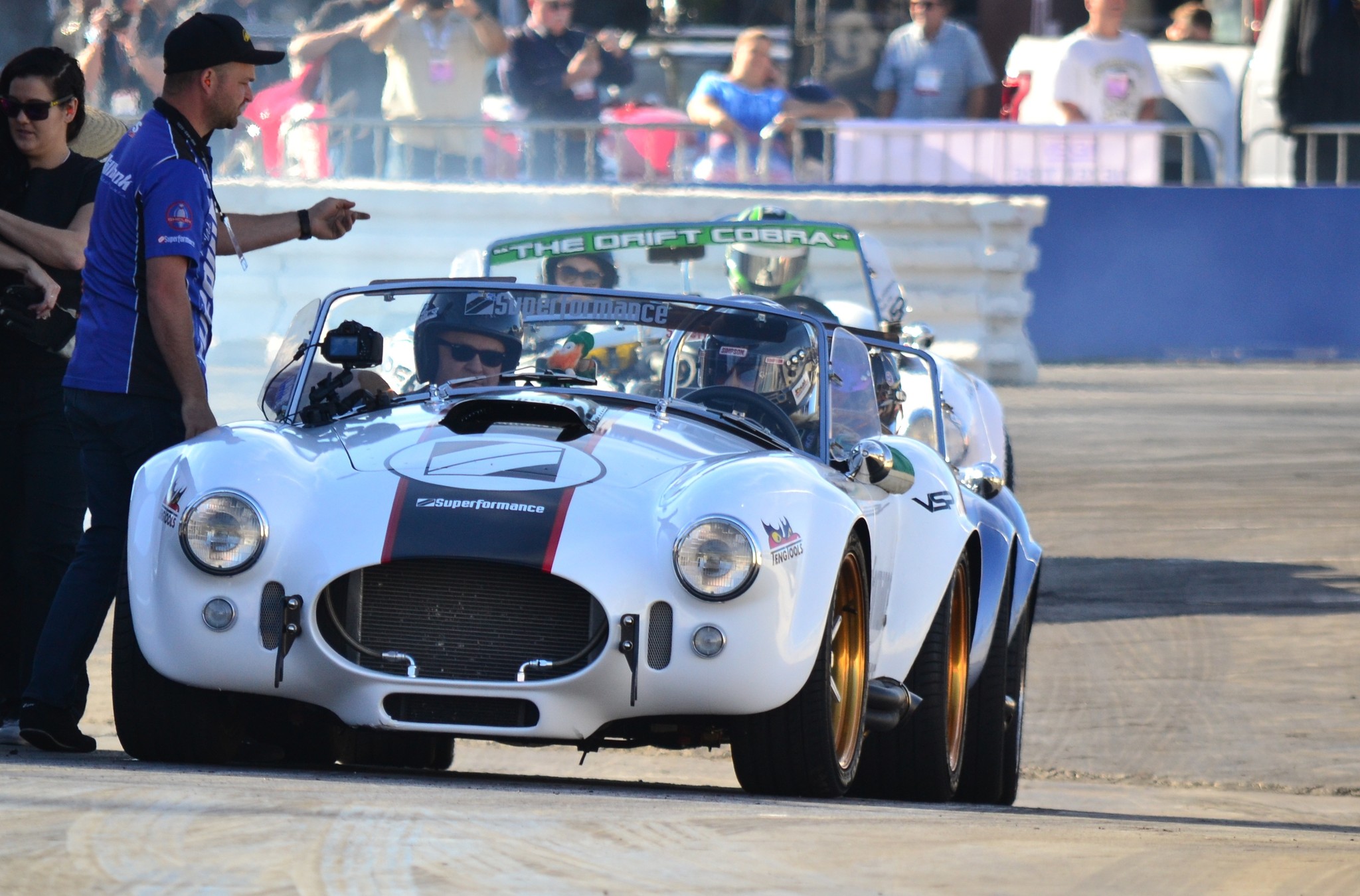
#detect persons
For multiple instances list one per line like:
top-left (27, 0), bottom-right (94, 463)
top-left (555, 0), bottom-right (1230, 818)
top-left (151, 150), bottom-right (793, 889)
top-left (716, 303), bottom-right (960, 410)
top-left (540, 249), bottom-right (620, 304)
top-left (826, 343), bottom-right (907, 435)
top-left (721, 202), bottom-right (812, 302)
top-left (15, 8), bottom-right (373, 756)
top-left (1, 0), bottom-right (1360, 192)
top-left (698, 294), bottom-right (863, 474)
top-left (263, 290), bottom-right (525, 425)
top-left (0, 45), bottom-right (107, 747)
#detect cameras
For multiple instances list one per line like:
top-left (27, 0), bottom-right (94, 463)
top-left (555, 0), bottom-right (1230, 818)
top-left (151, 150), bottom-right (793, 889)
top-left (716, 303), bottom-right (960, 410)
top-left (320, 320), bottom-right (385, 370)
top-left (103, 0), bottom-right (130, 31)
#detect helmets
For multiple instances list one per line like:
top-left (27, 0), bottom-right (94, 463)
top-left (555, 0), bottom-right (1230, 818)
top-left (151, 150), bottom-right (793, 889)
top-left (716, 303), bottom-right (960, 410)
top-left (697, 303), bottom-right (815, 417)
top-left (413, 286), bottom-right (523, 384)
top-left (1166, 2), bottom-right (1213, 40)
top-left (725, 204), bottom-right (808, 300)
top-left (542, 249), bottom-right (619, 291)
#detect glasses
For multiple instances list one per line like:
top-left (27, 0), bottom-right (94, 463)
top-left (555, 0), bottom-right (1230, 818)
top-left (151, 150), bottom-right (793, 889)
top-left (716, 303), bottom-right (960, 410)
top-left (3, 93), bottom-right (74, 121)
top-left (557, 265), bottom-right (604, 282)
top-left (540, 1), bottom-right (572, 11)
top-left (709, 360), bottom-right (770, 387)
top-left (434, 336), bottom-right (506, 368)
top-left (909, 1), bottom-right (940, 11)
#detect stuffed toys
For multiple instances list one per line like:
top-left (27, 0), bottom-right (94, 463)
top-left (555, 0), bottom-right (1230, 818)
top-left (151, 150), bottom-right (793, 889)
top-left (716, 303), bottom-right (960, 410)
top-left (540, 330), bottom-right (595, 388)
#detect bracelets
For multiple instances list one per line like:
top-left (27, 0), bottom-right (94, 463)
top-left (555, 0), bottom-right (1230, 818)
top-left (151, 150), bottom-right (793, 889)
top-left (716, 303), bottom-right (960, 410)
top-left (297, 208), bottom-right (312, 240)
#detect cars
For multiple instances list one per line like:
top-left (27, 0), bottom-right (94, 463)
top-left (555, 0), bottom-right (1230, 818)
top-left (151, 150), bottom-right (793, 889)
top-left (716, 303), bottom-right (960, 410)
top-left (109, 208), bottom-right (1044, 805)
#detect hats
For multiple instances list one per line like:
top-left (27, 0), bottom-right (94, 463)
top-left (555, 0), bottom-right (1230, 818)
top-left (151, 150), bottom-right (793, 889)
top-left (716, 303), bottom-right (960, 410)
top-left (67, 103), bottom-right (129, 160)
top-left (163, 12), bottom-right (286, 74)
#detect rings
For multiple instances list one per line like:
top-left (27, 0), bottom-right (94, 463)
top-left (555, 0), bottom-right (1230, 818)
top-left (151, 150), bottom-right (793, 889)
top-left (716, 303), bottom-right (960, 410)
top-left (50, 296), bottom-right (53, 298)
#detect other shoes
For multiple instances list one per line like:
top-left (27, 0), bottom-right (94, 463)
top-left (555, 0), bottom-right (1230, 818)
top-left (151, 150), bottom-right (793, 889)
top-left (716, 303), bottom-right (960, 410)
top-left (0, 719), bottom-right (30, 748)
top-left (19, 719), bottom-right (96, 754)
